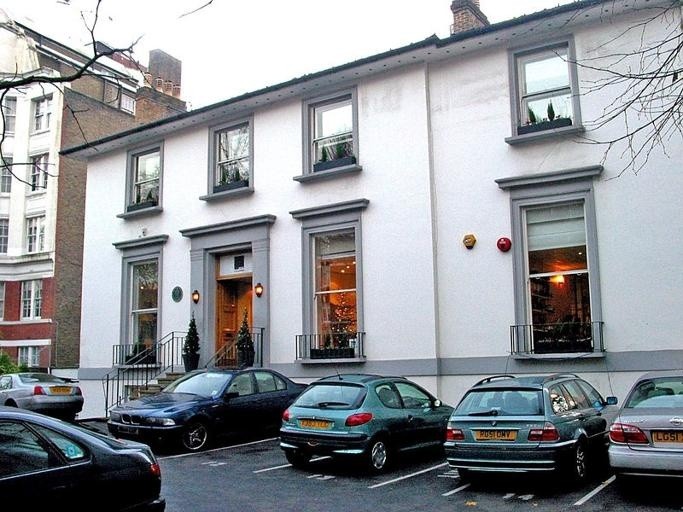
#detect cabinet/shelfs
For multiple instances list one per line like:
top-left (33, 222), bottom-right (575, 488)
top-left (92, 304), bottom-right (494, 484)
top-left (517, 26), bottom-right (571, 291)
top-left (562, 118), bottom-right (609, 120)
top-left (529, 278), bottom-right (550, 326)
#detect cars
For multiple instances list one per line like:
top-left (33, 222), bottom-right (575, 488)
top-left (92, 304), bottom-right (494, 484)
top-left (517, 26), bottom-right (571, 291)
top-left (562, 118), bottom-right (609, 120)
top-left (607, 370), bottom-right (683, 491)
top-left (107, 368), bottom-right (309, 452)
top-left (0, 371), bottom-right (85, 420)
top-left (0, 405), bottom-right (166, 511)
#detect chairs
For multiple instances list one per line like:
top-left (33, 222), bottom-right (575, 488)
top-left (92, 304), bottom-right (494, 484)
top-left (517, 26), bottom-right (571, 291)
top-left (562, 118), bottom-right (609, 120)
top-left (486, 391), bottom-right (525, 411)
top-left (377, 387), bottom-right (395, 407)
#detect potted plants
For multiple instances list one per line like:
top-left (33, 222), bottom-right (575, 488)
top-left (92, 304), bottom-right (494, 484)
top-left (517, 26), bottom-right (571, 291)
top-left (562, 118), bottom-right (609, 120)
top-left (236, 306), bottom-right (256, 368)
top-left (181, 309), bottom-right (201, 372)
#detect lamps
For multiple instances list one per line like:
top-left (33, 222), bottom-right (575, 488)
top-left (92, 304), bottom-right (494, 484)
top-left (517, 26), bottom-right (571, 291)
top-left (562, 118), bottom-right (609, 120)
top-left (191, 288), bottom-right (199, 305)
top-left (254, 282), bottom-right (262, 298)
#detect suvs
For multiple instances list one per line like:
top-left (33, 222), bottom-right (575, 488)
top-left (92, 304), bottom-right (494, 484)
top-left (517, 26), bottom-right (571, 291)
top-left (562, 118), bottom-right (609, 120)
top-left (279, 373), bottom-right (454, 473)
top-left (442, 372), bottom-right (620, 488)
